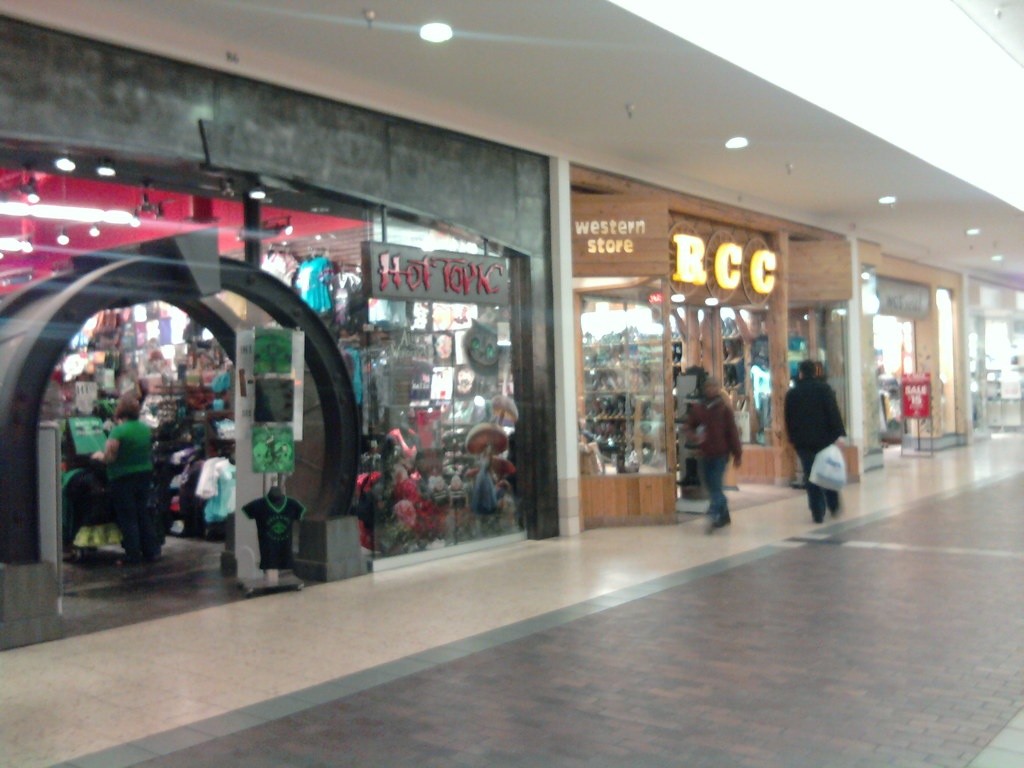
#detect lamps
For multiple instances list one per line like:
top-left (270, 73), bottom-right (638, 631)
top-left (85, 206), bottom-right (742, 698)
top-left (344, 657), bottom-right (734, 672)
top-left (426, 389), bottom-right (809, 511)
top-left (56, 227), bottom-right (70, 246)
top-left (27, 177), bottom-right (40, 204)
top-left (282, 224), bottom-right (293, 235)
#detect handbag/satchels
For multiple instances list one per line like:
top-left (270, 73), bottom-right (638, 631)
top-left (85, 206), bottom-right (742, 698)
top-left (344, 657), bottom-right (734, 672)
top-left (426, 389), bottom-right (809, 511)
top-left (808, 444), bottom-right (846, 492)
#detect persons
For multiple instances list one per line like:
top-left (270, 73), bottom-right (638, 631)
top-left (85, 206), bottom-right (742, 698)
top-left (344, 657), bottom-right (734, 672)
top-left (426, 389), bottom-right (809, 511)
top-left (684, 375), bottom-right (742, 535)
top-left (90, 395), bottom-right (158, 567)
top-left (783, 359), bottom-right (847, 521)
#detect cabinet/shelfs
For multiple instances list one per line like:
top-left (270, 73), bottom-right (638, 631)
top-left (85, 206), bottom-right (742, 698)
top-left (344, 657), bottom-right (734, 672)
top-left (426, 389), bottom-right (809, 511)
top-left (584, 336), bottom-right (746, 464)
top-left (199, 411), bottom-right (235, 541)
